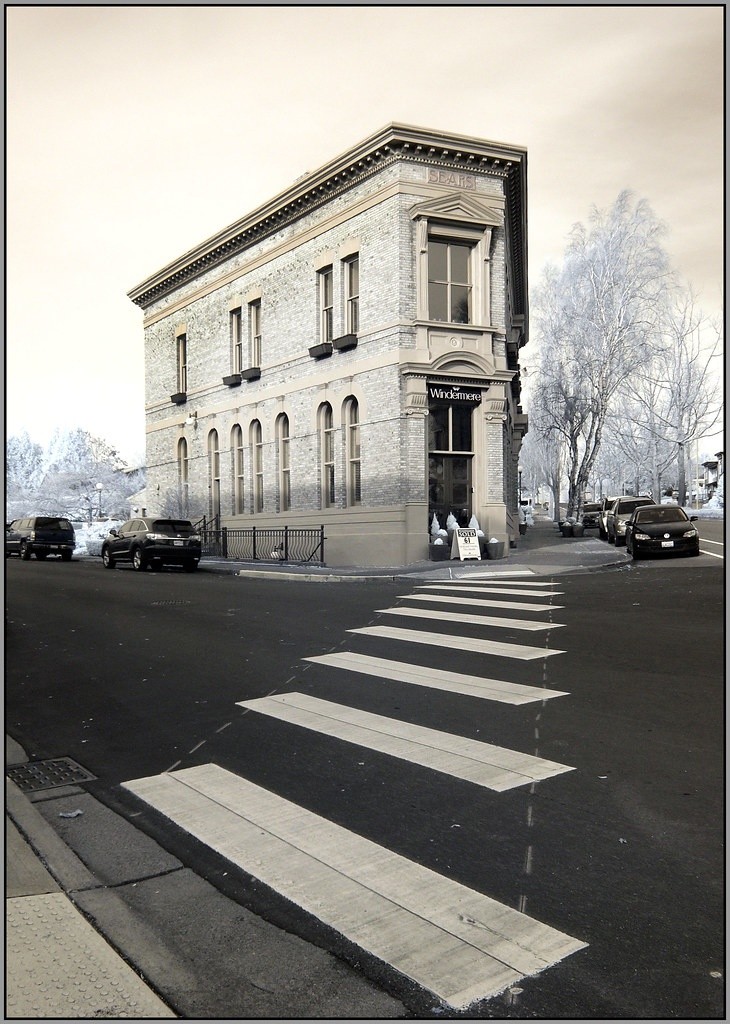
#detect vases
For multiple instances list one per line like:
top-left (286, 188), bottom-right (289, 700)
top-left (558, 521), bottom-right (585, 537)
top-left (428, 531), bottom-right (505, 562)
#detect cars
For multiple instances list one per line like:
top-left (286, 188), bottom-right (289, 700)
top-left (582, 493), bottom-right (702, 562)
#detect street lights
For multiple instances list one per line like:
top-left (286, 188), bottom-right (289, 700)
top-left (518, 465), bottom-right (522, 507)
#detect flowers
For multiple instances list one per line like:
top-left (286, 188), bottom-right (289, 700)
top-left (447, 511), bottom-right (460, 530)
top-left (563, 522), bottom-right (571, 526)
top-left (560, 516), bottom-right (567, 522)
top-left (430, 513), bottom-right (440, 535)
top-left (433, 537), bottom-right (444, 545)
top-left (572, 522), bottom-right (583, 526)
top-left (477, 530), bottom-right (485, 537)
top-left (436, 529), bottom-right (449, 536)
top-left (468, 515), bottom-right (480, 530)
top-left (489, 538), bottom-right (498, 543)
top-left (568, 517), bottom-right (576, 523)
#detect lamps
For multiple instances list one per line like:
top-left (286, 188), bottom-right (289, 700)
top-left (271, 542), bottom-right (283, 558)
top-left (186, 411), bottom-right (198, 425)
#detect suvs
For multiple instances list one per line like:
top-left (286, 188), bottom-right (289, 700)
top-left (6, 515), bottom-right (77, 561)
top-left (100, 518), bottom-right (203, 569)
